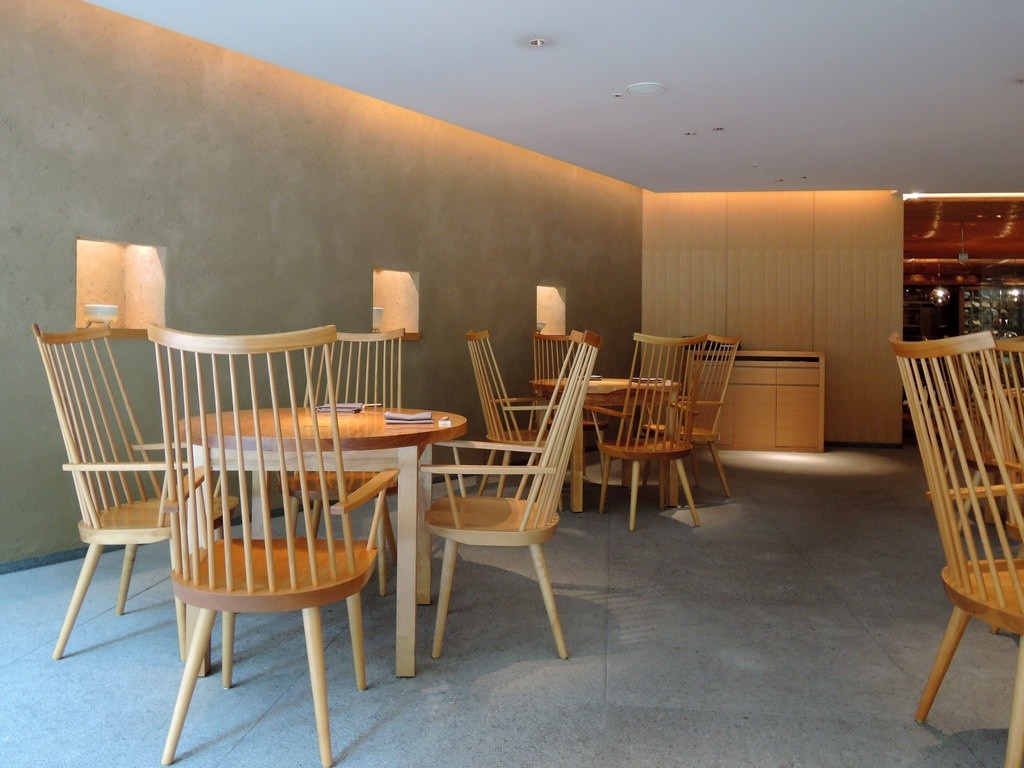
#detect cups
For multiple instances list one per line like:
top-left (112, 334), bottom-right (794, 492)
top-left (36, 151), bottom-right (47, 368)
top-left (373, 307), bottom-right (383, 332)
top-left (84, 305), bottom-right (118, 328)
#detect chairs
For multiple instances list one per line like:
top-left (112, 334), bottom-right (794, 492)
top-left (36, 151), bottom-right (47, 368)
top-left (146, 322), bottom-right (379, 768)
top-left (466, 327), bottom-right (742, 533)
top-left (272, 324), bottom-right (406, 565)
top-left (419, 328), bottom-right (604, 661)
top-left (886, 329), bottom-right (1024, 768)
top-left (32, 323), bottom-right (241, 666)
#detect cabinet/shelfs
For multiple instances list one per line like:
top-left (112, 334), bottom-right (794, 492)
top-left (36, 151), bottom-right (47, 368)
top-left (683, 348), bottom-right (826, 456)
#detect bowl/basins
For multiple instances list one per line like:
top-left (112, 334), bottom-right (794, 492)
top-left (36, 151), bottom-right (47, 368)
top-left (537, 323), bottom-right (547, 334)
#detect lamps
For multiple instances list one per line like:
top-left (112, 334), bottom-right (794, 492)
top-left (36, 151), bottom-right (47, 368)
top-left (372, 306), bottom-right (383, 334)
top-left (626, 82), bottom-right (667, 98)
top-left (957, 228), bottom-right (970, 263)
top-left (535, 320), bottom-right (548, 334)
top-left (930, 259), bottom-right (951, 307)
top-left (77, 304), bottom-right (121, 333)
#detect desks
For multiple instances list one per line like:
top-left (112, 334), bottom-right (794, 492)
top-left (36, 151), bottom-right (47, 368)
top-left (529, 379), bottom-right (684, 514)
top-left (174, 407), bottom-right (468, 676)
